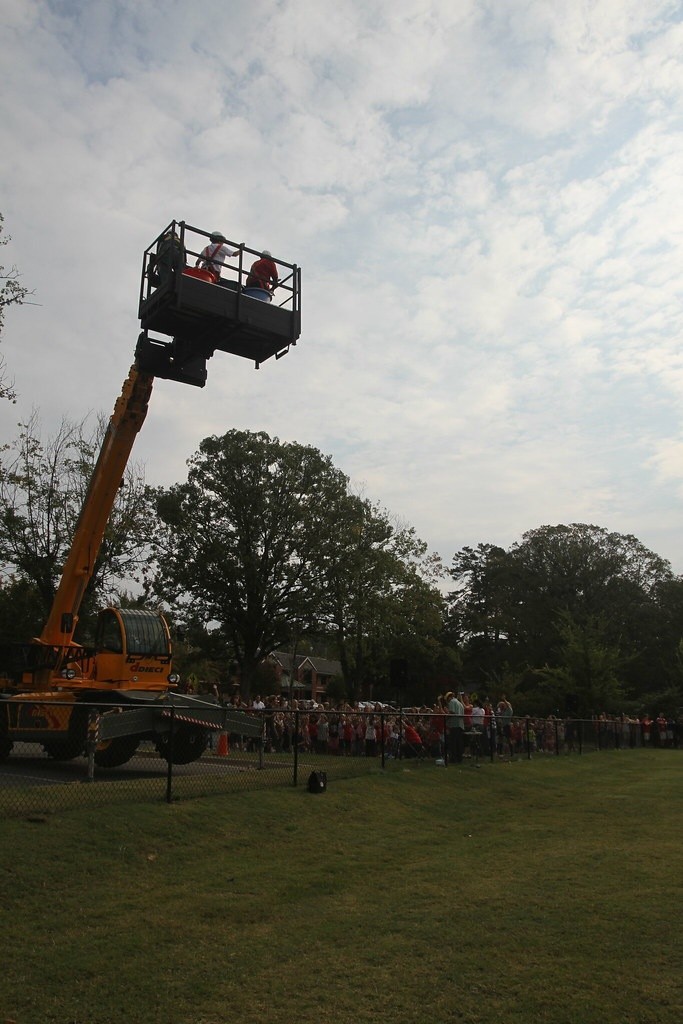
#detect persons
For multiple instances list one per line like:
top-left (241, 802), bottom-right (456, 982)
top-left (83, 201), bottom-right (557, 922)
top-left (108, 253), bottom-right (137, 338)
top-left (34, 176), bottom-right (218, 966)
top-left (182, 678), bottom-right (365, 757)
top-left (365, 698), bottom-right (448, 757)
top-left (246, 248), bottom-right (281, 296)
top-left (468, 695), bottom-right (573, 762)
top-left (590, 710), bottom-right (682, 749)
top-left (443, 692), bottom-right (464, 764)
top-left (458, 691), bottom-right (473, 756)
top-left (196, 230), bottom-right (245, 284)
top-left (155, 227), bottom-right (187, 285)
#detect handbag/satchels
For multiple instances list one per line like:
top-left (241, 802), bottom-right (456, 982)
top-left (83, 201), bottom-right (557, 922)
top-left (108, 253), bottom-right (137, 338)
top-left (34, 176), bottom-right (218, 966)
top-left (308, 768), bottom-right (328, 793)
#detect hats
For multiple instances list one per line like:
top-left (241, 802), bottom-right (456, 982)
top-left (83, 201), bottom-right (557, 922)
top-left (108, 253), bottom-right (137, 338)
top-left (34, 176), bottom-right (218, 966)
top-left (261, 249), bottom-right (271, 255)
top-left (444, 692), bottom-right (455, 700)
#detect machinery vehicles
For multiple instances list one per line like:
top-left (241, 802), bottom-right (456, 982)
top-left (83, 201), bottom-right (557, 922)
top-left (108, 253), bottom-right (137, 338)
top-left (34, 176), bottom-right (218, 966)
top-left (0, 218), bottom-right (303, 766)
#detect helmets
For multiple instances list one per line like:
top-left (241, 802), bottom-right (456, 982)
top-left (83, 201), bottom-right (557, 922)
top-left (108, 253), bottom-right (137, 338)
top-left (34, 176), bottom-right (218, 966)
top-left (167, 229), bottom-right (179, 237)
top-left (210, 231), bottom-right (226, 241)
